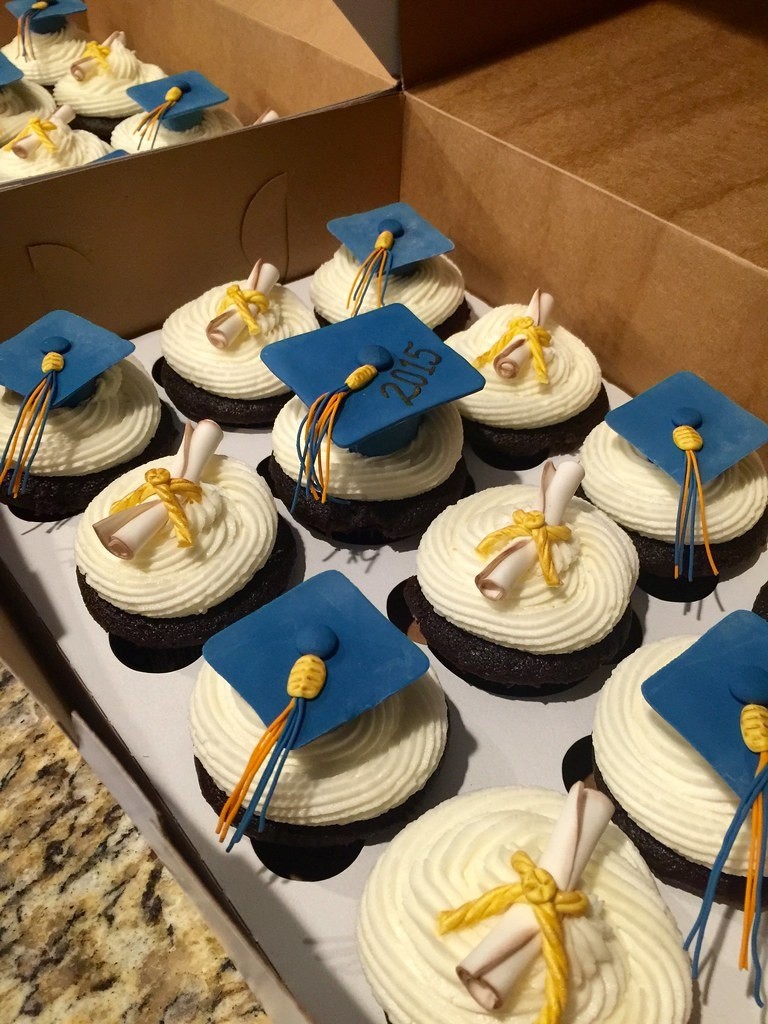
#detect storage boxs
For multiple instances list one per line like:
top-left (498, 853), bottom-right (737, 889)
top-left (0, 0), bottom-right (768, 1024)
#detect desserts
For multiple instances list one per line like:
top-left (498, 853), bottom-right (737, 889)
top-left (0, 0), bottom-right (277, 186)
top-left (0, 200), bottom-right (768, 1024)
